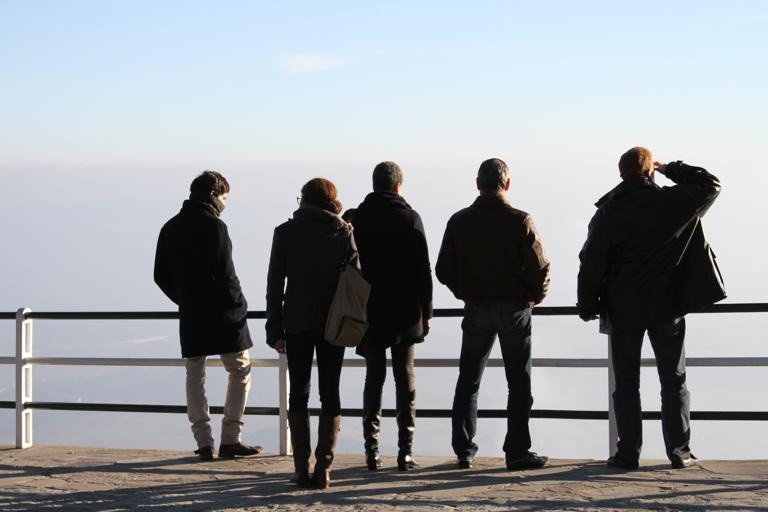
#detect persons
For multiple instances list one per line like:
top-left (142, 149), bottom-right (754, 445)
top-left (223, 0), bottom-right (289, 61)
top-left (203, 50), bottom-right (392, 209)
top-left (154, 171), bottom-right (263, 461)
top-left (576, 145), bottom-right (728, 471)
top-left (435, 158), bottom-right (551, 468)
top-left (341, 161), bottom-right (433, 470)
top-left (266, 178), bottom-right (361, 488)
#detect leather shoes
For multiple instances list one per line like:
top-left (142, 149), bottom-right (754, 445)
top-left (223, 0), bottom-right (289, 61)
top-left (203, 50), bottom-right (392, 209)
top-left (365, 452), bottom-right (383, 471)
top-left (672, 453), bottom-right (698, 468)
top-left (218, 442), bottom-right (262, 457)
top-left (607, 456), bottom-right (639, 470)
top-left (397, 454), bottom-right (419, 471)
top-left (193, 447), bottom-right (214, 461)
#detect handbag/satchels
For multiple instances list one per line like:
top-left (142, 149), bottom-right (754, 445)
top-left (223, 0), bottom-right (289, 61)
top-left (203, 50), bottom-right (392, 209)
top-left (326, 259), bottom-right (371, 348)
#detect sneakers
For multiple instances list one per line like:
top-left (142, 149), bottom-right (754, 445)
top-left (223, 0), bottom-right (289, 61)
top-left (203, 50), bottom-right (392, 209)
top-left (506, 452), bottom-right (548, 470)
top-left (456, 456), bottom-right (476, 469)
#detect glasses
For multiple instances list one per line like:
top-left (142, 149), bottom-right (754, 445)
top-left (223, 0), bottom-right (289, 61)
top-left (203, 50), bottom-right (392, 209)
top-left (297, 195), bottom-right (303, 205)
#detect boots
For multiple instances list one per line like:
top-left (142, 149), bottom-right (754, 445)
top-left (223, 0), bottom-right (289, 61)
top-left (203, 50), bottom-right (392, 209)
top-left (309, 414), bottom-right (340, 488)
top-left (283, 412), bottom-right (311, 489)
